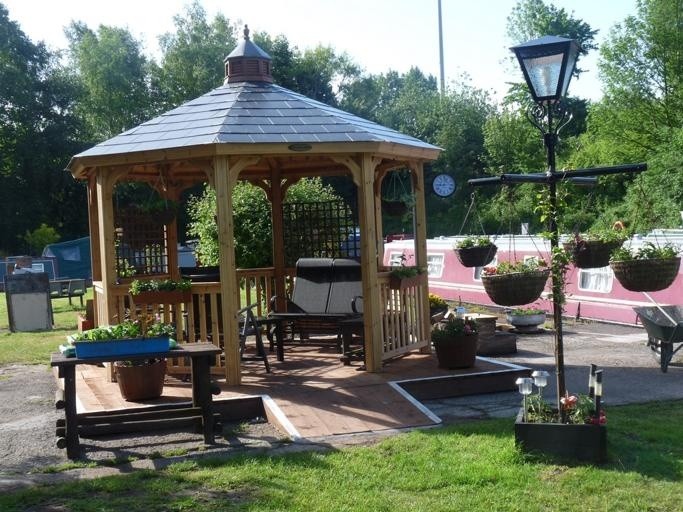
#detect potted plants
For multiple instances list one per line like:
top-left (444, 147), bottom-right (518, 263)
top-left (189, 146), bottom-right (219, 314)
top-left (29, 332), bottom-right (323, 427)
top-left (129, 278), bottom-right (192, 305)
top-left (451, 226), bottom-right (682, 333)
top-left (386, 262), bottom-right (428, 291)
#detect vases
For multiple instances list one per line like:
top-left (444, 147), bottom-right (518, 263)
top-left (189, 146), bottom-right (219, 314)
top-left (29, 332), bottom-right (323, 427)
top-left (72, 336), bottom-right (170, 358)
top-left (112, 362), bottom-right (166, 402)
top-left (433, 338), bottom-right (477, 368)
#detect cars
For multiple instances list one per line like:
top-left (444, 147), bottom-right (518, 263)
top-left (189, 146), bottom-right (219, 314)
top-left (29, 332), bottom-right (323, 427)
top-left (339, 232), bottom-right (378, 265)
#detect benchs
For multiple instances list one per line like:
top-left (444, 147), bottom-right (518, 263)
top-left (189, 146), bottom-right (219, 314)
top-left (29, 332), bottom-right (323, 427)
top-left (48, 278), bottom-right (86, 307)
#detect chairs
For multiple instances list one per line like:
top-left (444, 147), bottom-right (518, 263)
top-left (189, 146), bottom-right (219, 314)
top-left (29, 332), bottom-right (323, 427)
top-left (265, 257), bottom-right (365, 368)
top-left (179, 264), bottom-right (263, 382)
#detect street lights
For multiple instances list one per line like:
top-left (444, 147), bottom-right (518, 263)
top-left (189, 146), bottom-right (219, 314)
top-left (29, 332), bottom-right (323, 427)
top-left (508, 30), bottom-right (612, 466)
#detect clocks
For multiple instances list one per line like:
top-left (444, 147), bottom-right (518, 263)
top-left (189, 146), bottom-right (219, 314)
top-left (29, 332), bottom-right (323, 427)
top-left (431, 173), bottom-right (457, 198)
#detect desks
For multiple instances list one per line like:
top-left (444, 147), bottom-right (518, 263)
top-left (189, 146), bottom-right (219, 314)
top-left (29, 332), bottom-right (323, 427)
top-left (338, 311), bottom-right (411, 366)
top-left (237, 316), bottom-right (286, 361)
top-left (49, 342), bottom-right (222, 459)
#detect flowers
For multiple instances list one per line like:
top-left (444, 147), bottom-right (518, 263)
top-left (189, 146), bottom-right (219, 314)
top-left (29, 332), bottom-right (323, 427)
top-left (70, 312), bottom-right (175, 341)
top-left (513, 392), bottom-right (609, 424)
top-left (429, 312), bottom-right (478, 336)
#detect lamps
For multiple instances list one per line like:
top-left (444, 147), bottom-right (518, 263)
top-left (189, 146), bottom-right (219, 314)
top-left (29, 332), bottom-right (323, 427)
top-left (587, 363), bottom-right (603, 418)
top-left (513, 369), bottom-right (550, 422)
